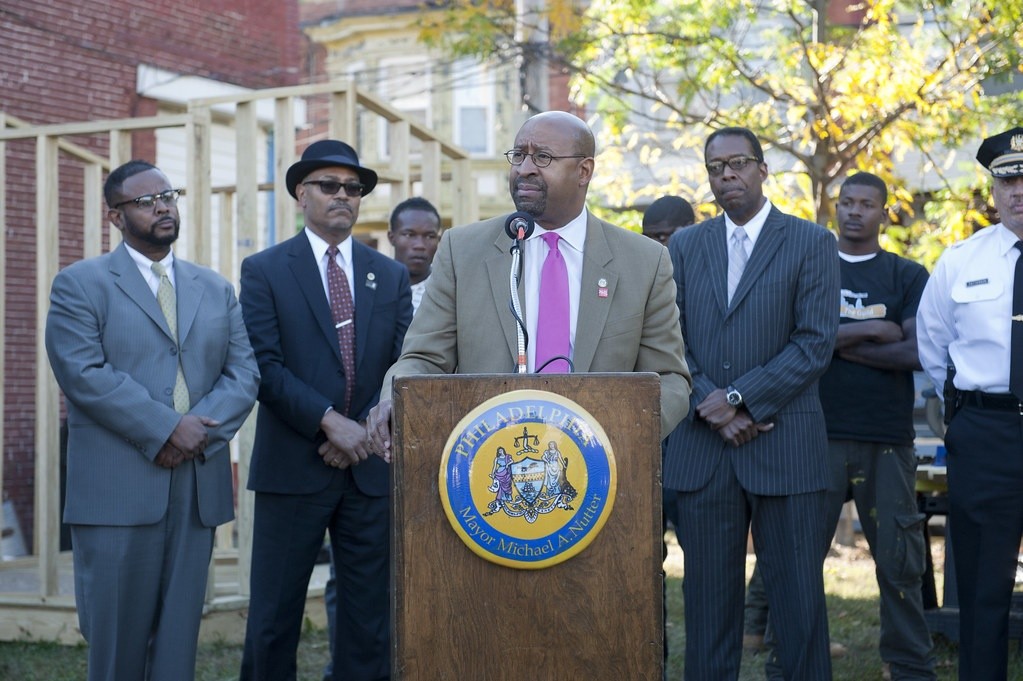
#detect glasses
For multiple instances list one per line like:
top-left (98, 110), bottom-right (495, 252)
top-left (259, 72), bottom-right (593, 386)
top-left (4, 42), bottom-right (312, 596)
top-left (302, 181), bottom-right (365, 198)
top-left (115, 188), bottom-right (186, 211)
top-left (705, 156), bottom-right (759, 172)
top-left (504, 149), bottom-right (586, 168)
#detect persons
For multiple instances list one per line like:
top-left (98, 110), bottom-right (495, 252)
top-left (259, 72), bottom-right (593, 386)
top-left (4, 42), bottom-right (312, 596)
top-left (323, 196), bottom-right (441, 681)
top-left (43, 160), bottom-right (261, 681)
top-left (238, 139), bottom-right (415, 681)
top-left (642, 172), bottom-right (937, 681)
top-left (915, 125), bottom-right (1023, 681)
top-left (364, 111), bottom-right (693, 464)
top-left (664, 127), bottom-right (843, 681)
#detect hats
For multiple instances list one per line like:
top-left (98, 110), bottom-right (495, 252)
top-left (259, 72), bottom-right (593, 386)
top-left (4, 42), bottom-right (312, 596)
top-left (974, 127), bottom-right (1023, 178)
top-left (285, 140), bottom-right (378, 202)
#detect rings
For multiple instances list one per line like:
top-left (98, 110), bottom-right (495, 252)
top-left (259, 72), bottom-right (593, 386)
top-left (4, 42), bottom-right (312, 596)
top-left (334, 459), bottom-right (340, 464)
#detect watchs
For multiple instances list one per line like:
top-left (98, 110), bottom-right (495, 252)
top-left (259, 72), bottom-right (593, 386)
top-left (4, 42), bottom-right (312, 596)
top-left (726, 385), bottom-right (743, 408)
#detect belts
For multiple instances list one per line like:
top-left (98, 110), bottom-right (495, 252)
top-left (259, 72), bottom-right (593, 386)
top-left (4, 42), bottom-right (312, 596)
top-left (946, 386), bottom-right (1022, 411)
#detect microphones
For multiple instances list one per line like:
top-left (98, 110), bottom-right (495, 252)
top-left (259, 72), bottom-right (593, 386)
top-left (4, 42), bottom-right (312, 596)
top-left (505, 211), bottom-right (535, 240)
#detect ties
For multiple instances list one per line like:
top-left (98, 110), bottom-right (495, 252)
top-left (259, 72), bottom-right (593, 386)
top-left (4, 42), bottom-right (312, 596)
top-left (536, 232), bottom-right (570, 374)
top-left (728, 227), bottom-right (750, 311)
top-left (325, 246), bottom-right (355, 417)
top-left (151, 262), bottom-right (190, 417)
top-left (1010, 240), bottom-right (1023, 400)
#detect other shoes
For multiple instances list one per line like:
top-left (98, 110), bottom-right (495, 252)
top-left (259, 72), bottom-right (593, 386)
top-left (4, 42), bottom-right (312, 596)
top-left (744, 633), bottom-right (768, 657)
top-left (830, 641), bottom-right (845, 659)
